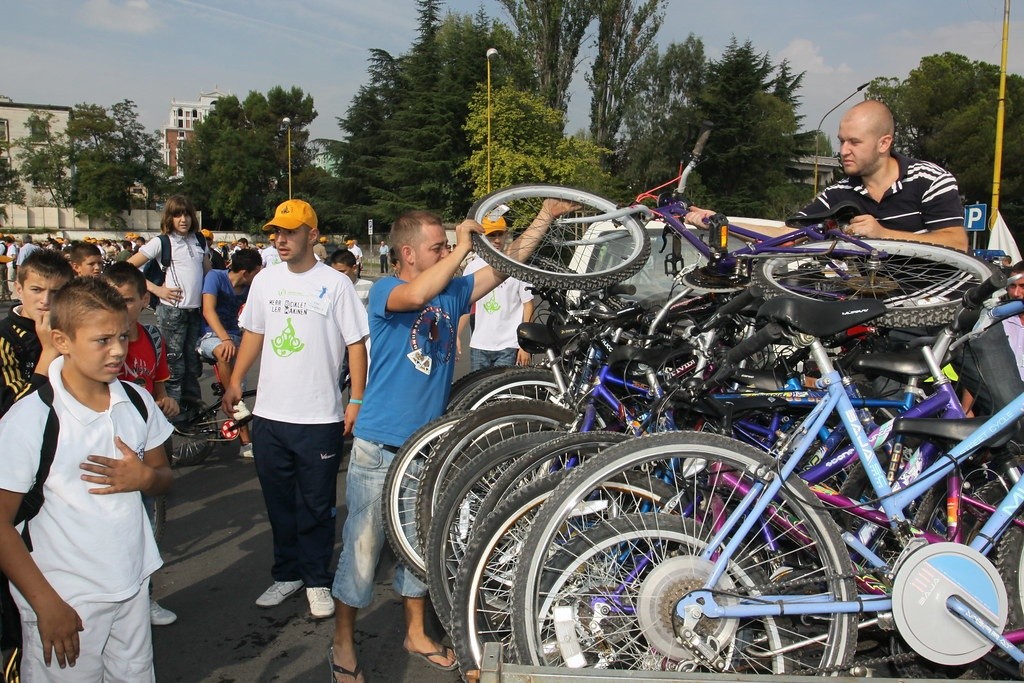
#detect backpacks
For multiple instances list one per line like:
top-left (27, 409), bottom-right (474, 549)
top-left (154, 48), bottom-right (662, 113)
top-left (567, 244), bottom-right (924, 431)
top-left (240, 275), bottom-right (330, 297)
top-left (144, 230), bottom-right (207, 309)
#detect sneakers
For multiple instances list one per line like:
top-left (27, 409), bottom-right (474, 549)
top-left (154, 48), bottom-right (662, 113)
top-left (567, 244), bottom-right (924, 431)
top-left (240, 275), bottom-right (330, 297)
top-left (149, 599), bottom-right (177, 625)
top-left (255, 578), bottom-right (305, 605)
top-left (306, 586), bottom-right (335, 617)
top-left (239, 442), bottom-right (254, 458)
top-left (232, 400), bottom-right (249, 420)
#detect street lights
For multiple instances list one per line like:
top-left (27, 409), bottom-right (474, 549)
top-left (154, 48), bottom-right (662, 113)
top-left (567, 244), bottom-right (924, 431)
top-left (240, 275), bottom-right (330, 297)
top-left (814, 80), bottom-right (871, 196)
top-left (486, 48), bottom-right (499, 194)
top-left (283, 118), bottom-right (292, 200)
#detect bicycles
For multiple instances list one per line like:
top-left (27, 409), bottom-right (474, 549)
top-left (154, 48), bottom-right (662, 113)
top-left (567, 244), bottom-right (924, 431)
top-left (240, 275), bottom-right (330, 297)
top-left (142, 406), bottom-right (167, 550)
top-left (381, 253), bottom-right (1023, 676)
top-left (466, 121), bottom-right (1006, 331)
top-left (171, 354), bottom-right (257, 467)
top-left (340, 348), bottom-right (352, 412)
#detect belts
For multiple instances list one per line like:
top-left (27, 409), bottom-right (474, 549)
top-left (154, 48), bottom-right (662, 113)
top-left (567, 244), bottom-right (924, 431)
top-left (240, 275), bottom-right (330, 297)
top-left (370, 441), bottom-right (427, 459)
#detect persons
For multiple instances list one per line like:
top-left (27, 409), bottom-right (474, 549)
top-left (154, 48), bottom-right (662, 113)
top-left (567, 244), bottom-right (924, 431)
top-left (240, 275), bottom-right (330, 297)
top-left (0, 274), bottom-right (175, 683)
top-left (223, 199), bottom-right (370, 618)
top-left (327, 197), bottom-right (582, 683)
top-left (0, 249), bottom-right (75, 683)
top-left (0, 195), bottom-right (269, 457)
top-left (97, 261), bottom-right (182, 625)
top-left (684, 101), bottom-right (1024, 461)
top-left (313, 212), bottom-right (533, 399)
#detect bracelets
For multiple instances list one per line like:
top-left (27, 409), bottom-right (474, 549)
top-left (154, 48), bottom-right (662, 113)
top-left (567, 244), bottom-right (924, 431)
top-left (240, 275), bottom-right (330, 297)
top-left (349, 399), bottom-right (363, 404)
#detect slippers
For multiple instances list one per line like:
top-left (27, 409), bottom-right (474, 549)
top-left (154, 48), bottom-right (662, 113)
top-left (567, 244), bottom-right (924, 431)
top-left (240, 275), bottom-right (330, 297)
top-left (403, 644), bottom-right (458, 670)
top-left (327, 647), bottom-right (363, 683)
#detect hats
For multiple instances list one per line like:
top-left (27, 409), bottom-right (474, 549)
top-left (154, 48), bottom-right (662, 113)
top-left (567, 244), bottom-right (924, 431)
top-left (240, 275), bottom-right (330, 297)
top-left (0, 232), bottom-right (138, 249)
top-left (201, 228), bottom-right (276, 248)
top-left (346, 239), bottom-right (354, 244)
top-left (481, 213), bottom-right (508, 235)
top-left (261, 199), bottom-right (317, 231)
top-left (319, 237), bottom-right (328, 242)
